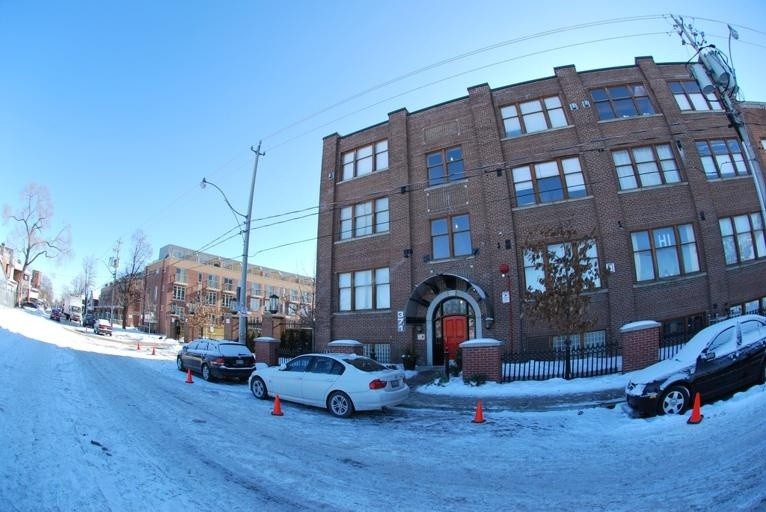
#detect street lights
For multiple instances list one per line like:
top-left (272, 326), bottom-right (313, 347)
top-left (199, 138), bottom-right (265, 342)
top-left (661, 12), bottom-right (766, 232)
top-left (95, 234), bottom-right (123, 323)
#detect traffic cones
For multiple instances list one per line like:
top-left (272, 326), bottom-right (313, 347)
top-left (136, 342), bottom-right (141, 350)
top-left (687, 392), bottom-right (703, 424)
top-left (151, 344), bottom-right (156, 355)
top-left (470, 399), bottom-right (486, 424)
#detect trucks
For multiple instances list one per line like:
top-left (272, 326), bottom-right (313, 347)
top-left (63, 295), bottom-right (82, 322)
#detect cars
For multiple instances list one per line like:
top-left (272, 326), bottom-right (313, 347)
top-left (50, 309), bottom-right (60, 321)
top-left (83, 314), bottom-right (112, 336)
top-left (626, 314), bottom-right (766, 419)
top-left (177, 338), bottom-right (409, 419)
